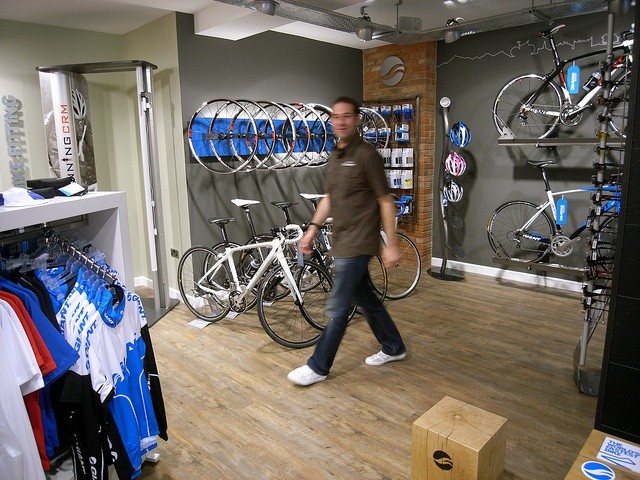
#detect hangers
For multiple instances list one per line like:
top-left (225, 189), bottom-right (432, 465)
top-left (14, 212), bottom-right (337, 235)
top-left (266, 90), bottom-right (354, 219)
top-left (9, 227), bottom-right (124, 301)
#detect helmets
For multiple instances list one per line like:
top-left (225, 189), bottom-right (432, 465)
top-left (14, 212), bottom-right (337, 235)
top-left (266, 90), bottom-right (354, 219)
top-left (443, 175), bottom-right (464, 203)
top-left (446, 151), bottom-right (467, 178)
top-left (71, 89), bottom-right (87, 119)
top-left (450, 122), bottom-right (472, 148)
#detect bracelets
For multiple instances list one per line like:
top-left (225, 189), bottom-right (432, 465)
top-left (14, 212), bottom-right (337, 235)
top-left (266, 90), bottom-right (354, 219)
top-left (308, 222), bottom-right (321, 229)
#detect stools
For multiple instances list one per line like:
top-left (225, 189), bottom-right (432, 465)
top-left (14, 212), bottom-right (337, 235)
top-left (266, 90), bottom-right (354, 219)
top-left (413, 395), bottom-right (508, 478)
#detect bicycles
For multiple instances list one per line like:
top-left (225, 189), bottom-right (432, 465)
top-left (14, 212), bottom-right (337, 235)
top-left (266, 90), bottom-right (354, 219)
top-left (487, 159), bottom-right (623, 275)
top-left (490, 23), bottom-right (635, 140)
top-left (178, 192), bottom-right (422, 349)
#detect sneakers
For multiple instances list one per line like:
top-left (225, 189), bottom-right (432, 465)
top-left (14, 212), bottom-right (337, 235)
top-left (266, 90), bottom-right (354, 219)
top-left (365, 350), bottom-right (406, 366)
top-left (286, 364), bottom-right (328, 386)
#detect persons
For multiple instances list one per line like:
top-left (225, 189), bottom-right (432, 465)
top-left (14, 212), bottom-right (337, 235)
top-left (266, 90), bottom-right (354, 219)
top-left (286, 97), bottom-right (406, 386)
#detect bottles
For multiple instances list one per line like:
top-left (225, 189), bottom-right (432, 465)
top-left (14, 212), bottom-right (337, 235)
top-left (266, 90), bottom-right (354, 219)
top-left (583, 71), bottom-right (603, 93)
top-left (244, 259), bottom-right (261, 279)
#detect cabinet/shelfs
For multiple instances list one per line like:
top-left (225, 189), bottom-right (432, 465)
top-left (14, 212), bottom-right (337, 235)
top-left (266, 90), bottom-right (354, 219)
top-left (493, 135), bottom-right (623, 277)
